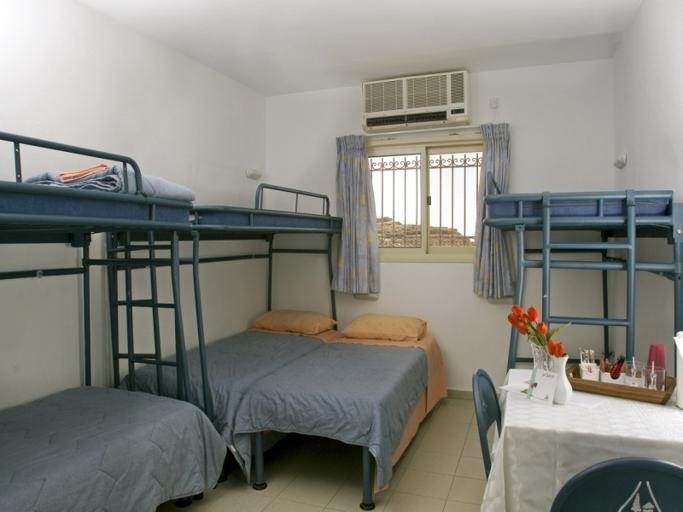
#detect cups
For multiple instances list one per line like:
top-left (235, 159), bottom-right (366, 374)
top-left (624, 361), bottom-right (645, 388)
top-left (642, 366), bottom-right (666, 393)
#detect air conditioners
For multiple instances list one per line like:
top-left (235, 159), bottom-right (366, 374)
top-left (360, 69), bottom-right (473, 135)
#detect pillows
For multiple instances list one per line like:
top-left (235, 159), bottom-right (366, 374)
top-left (253, 308), bottom-right (336, 336)
top-left (344, 312), bottom-right (428, 342)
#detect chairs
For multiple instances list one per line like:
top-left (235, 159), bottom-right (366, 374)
top-left (473, 368), bottom-right (503, 478)
top-left (547, 456), bottom-right (682, 512)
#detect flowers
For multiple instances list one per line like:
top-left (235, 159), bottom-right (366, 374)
top-left (506, 303), bottom-right (572, 356)
top-left (547, 341), bottom-right (566, 357)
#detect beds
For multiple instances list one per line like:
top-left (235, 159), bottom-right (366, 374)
top-left (243, 315), bottom-right (448, 511)
top-left (121, 309), bottom-right (343, 482)
top-left (1, 131), bottom-right (195, 405)
top-left (0, 383), bottom-right (228, 511)
top-left (481, 172), bottom-right (683, 375)
top-left (106, 183), bottom-right (343, 422)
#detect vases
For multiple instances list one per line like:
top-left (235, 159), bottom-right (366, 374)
top-left (547, 356), bottom-right (574, 405)
top-left (527, 341), bottom-right (547, 398)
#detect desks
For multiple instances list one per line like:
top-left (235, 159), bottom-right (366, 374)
top-left (482, 366), bottom-right (682, 511)
